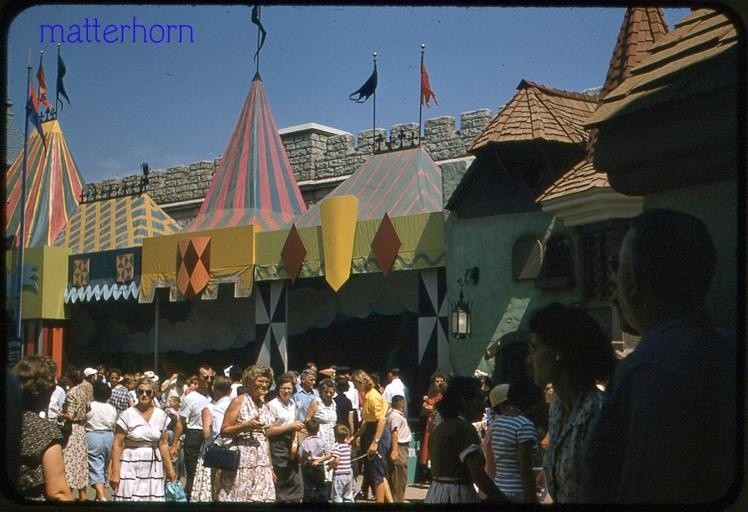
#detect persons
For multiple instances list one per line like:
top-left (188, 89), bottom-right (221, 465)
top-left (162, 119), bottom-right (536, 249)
top-left (528, 301), bottom-right (618, 504)
top-left (578, 206), bottom-right (737, 502)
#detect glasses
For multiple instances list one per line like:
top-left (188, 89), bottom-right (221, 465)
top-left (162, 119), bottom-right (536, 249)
top-left (200, 376), bottom-right (215, 381)
top-left (136, 390), bottom-right (153, 395)
top-left (608, 254), bottom-right (632, 275)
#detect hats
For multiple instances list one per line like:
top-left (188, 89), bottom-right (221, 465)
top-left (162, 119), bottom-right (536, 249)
top-left (141, 371), bottom-right (159, 382)
top-left (84, 368), bottom-right (98, 377)
top-left (489, 383), bottom-right (511, 408)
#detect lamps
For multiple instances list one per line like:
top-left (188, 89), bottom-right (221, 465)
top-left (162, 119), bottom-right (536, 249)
top-left (445, 265), bottom-right (479, 340)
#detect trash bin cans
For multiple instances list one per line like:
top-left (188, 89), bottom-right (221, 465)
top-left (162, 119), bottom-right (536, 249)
top-left (407, 417), bottom-right (421, 484)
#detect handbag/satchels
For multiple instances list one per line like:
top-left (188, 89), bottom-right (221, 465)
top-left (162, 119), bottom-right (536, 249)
top-left (56, 419), bottom-right (71, 450)
top-left (203, 432), bottom-right (240, 471)
top-left (164, 479), bottom-right (188, 502)
